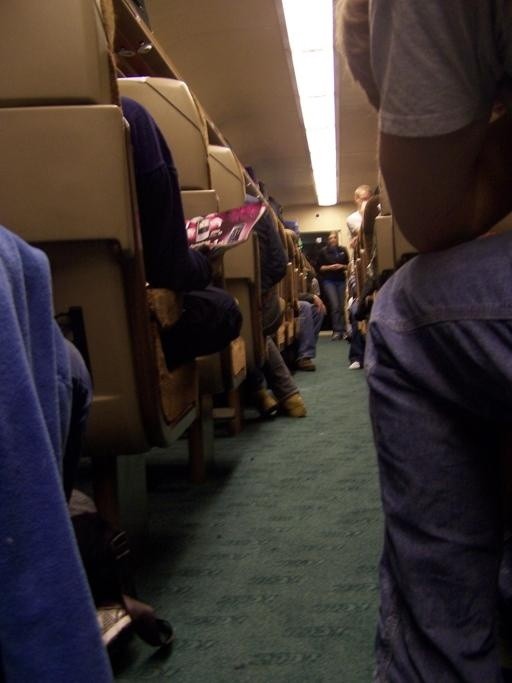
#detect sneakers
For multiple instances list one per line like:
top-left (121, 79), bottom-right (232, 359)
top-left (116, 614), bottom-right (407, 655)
top-left (295, 356), bottom-right (317, 372)
top-left (280, 394), bottom-right (307, 418)
top-left (249, 387), bottom-right (279, 417)
top-left (330, 331), bottom-right (341, 340)
top-left (347, 361), bottom-right (361, 370)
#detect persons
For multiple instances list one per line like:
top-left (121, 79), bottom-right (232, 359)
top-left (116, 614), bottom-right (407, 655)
top-left (363, 1), bottom-right (510, 681)
top-left (317, 227), bottom-right (352, 342)
top-left (334, 0), bottom-right (381, 110)
top-left (345, 174), bottom-right (410, 371)
top-left (246, 194), bottom-right (327, 418)
top-left (99, 0), bottom-right (244, 360)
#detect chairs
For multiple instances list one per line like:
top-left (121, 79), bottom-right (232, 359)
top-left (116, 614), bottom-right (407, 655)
top-left (346, 168), bottom-right (416, 339)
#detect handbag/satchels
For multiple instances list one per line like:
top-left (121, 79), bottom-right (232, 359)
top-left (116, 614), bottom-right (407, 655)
top-left (69, 493), bottom-right (176, 674)
